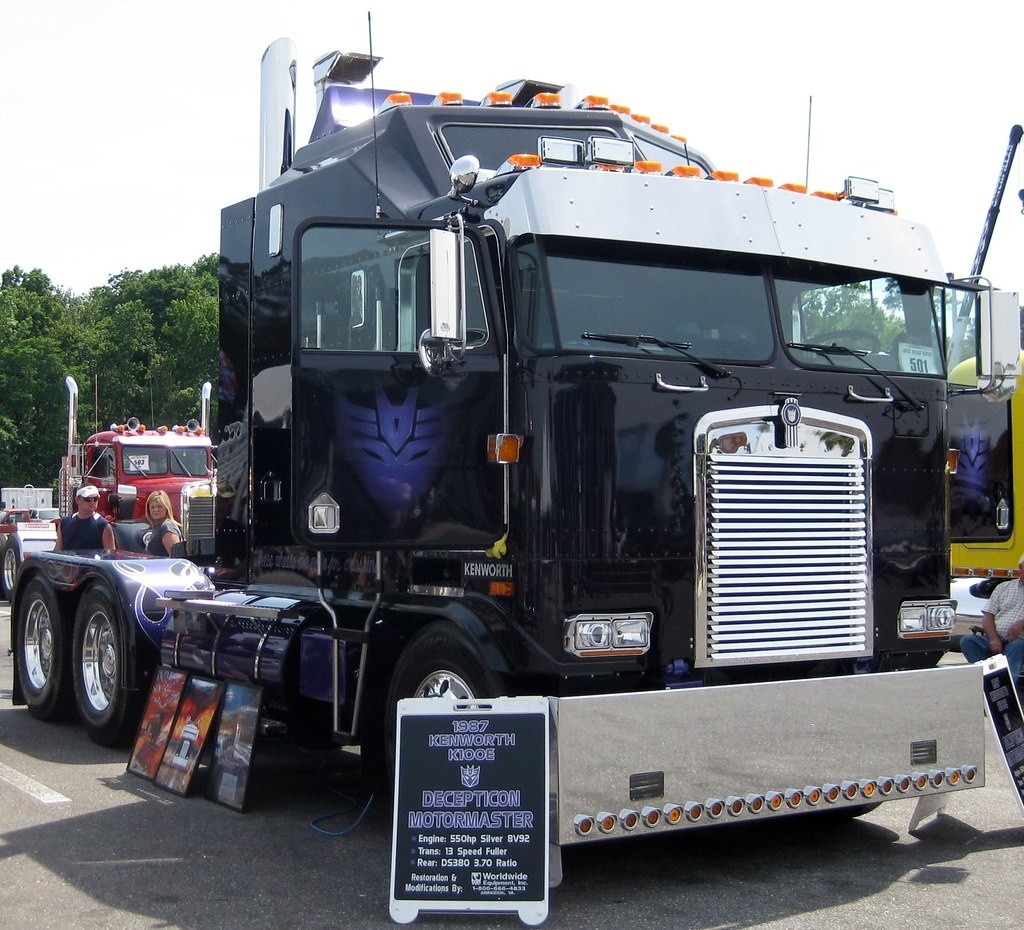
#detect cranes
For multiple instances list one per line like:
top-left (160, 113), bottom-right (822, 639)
top-left (939, 118), bottom-right (1023, 382)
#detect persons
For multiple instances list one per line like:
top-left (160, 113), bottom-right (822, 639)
top-left (54, 481), bottom-right (117, 552)
top-left (959, 554), bottom-right (1023, 683)
top-left (145, 490), bottom-right (183, 557)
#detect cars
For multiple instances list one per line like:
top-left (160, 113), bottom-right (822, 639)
top-left (28, 508), bottom-right (63, 522)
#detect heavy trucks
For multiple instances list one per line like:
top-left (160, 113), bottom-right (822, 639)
top-left (10, 29), bottom-right (992, 848)
top-left (0, 374), bottom-right (217, 602)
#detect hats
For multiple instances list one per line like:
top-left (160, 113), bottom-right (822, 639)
top-left (76, 485), bottom-right (100, 497)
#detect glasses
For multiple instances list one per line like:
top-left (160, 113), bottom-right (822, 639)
top-left (79, 496), bottom-right (98, 503)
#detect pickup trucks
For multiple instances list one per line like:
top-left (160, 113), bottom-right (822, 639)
top-left (0, 509), bottom-right (31, 534)
